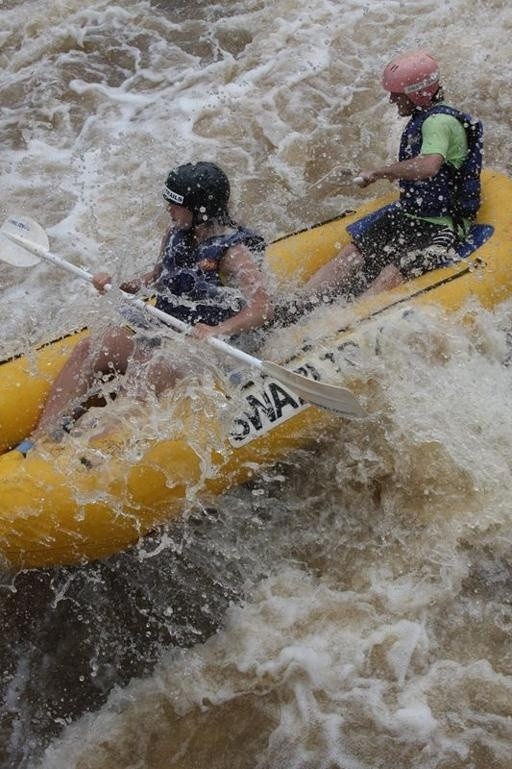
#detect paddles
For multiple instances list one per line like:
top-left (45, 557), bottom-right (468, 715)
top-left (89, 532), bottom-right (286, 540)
top-left (0, 215), bottom-right (369, 419)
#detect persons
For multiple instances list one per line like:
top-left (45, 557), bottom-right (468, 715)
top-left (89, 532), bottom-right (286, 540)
top-left (8, 161), bottom-right (275, 452)
top-left (276, 50), bottom-right (483, 321)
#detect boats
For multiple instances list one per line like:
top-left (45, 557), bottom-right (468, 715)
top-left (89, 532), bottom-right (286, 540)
top-left (1, 164), bottom-right (512, 582)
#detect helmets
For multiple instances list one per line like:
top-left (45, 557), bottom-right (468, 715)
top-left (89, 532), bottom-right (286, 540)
top-left (382, 53), bottom-right (440, 107)
top-left (161, 161), bottom-right (230, 214)
